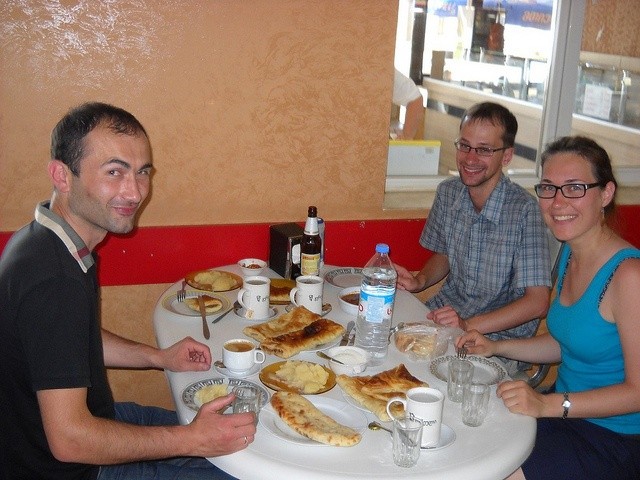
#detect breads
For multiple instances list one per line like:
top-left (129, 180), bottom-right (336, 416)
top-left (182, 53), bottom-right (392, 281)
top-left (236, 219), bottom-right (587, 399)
top-left (269, 285), bottom-right (293, 302)
top-left (336, 363), bottom-right (430, 422)
top-left (185, 295), bottom-right (222, 313)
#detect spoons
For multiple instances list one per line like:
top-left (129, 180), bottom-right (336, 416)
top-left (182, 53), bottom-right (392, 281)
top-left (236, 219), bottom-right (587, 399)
top-left (210, 301), bottom-right (243, 325)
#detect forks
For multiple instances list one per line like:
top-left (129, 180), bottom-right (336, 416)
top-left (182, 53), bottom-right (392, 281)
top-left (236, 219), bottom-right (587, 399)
top-left (177, 280), bottom-right (187, 302)
top-left (458, 343), bottom-right (468, 359)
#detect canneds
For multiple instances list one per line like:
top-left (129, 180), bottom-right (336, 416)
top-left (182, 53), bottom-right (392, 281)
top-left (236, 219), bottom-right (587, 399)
top-left (317, 218), bottom-right (324, 262)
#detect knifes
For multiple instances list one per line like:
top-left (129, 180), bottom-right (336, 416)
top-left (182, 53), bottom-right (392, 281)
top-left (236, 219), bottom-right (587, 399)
top-left (340, 319), bottom-right (355, 347)
top-left (198, 292), bottom-right (211, 340)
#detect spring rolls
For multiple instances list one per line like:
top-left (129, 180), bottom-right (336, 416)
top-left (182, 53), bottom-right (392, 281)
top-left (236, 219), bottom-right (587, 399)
top-left (242, 305), bottom-right (322, 342)
top-left (270, 390), bottom-right (362, 447)
top-left (260, 319), bottom-right (345, 359)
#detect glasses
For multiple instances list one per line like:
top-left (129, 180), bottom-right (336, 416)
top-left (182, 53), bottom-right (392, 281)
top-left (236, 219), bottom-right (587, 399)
top-left (454, 135), bottom-right (509, 156)
top-left (535, 182), bottom-right (602, 199)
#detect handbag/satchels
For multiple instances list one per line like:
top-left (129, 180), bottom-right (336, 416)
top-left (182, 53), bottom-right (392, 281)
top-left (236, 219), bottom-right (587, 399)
top-left (489, 11), bottom-right (505, 51)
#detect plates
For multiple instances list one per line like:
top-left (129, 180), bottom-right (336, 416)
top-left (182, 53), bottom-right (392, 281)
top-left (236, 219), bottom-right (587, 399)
top-left (324, 268), bottom-right (363, 288)
top-left (428, 354), bottom-right (508, 391)
top-left (161, 290), bottom-right (232, 317)
top-left (182, 377), bottom-right (271, 414)
top-left (259, 361), bottom-right (337, 395)
top-left (340, 386), bottom-right (373, 415)
top-left (257, 394), bottom-right (369, 446)
top-left (214, 353), bottom-right (260, 377)
top-left (184, 269), bottom-right (244, 292)
top-left (419, 421), bottom-right (457, 453)
top-left (234, 304), bottom-right (279, 320)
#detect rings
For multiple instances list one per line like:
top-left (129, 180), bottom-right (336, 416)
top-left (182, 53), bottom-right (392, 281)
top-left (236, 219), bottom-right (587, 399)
top-left (244, 437), bottom-right (248, 445)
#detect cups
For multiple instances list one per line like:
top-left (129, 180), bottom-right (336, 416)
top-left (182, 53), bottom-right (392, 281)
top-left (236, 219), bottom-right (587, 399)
top-left (289, 274), bottom-right (325, 316)
top-left (232, 386), bottom-right (261, 426)
top-left (238, 278), bottom-right (271, 320)
top-left (386, 387), bottom-right (445, 448)
top-left (462, 383), bottom-right (489, 428)
top-left (447, 359), bottom-right (475, 403)
top-left (221, 337), bottom-right (265, 375)
top-left (392, 416), bottom-right (421, 468)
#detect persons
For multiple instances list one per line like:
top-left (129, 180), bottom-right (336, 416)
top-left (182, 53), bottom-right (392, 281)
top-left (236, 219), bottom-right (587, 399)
top-left (371, 102), bottom-right (552, 372)
top-left (0, 101), bottom-right (256, 480)
top-left (454, 135), bottom-right (640, 480)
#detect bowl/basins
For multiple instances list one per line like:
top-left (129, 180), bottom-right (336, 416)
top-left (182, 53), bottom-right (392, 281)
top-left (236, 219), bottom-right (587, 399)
top-left (338, 286), bottom-right (364, 316)
top-left (237, 257), bottom-right (268, 277)
top-left (329, 346), bottom-right (369, 375)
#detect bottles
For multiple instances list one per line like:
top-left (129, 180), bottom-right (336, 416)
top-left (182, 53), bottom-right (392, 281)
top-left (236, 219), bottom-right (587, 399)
top-left (354, 244), bottom-right (399, 360)
top-left (299, 206), bottom-right (322, 277)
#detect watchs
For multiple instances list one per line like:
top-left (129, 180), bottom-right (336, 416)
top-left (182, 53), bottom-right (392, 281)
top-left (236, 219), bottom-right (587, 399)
top-left (562, 394), bottom-right (570, 419)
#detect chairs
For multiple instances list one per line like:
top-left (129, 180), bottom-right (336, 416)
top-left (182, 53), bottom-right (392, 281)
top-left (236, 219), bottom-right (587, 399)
top-left (520, 283), bottom-right (561, 395)
